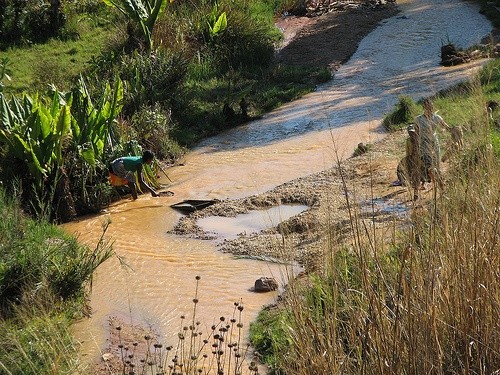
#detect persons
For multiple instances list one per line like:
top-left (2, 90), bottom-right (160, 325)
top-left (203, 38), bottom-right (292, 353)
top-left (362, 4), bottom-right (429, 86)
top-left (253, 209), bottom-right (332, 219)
top-left (110, 151), bottom-right (160, 201)
top-left (412, 96), bottom-right (464, 192)
top-left (443, 97), bottom-right (500, 161)
top-left (404, 123), bottom-right (427, 203)
